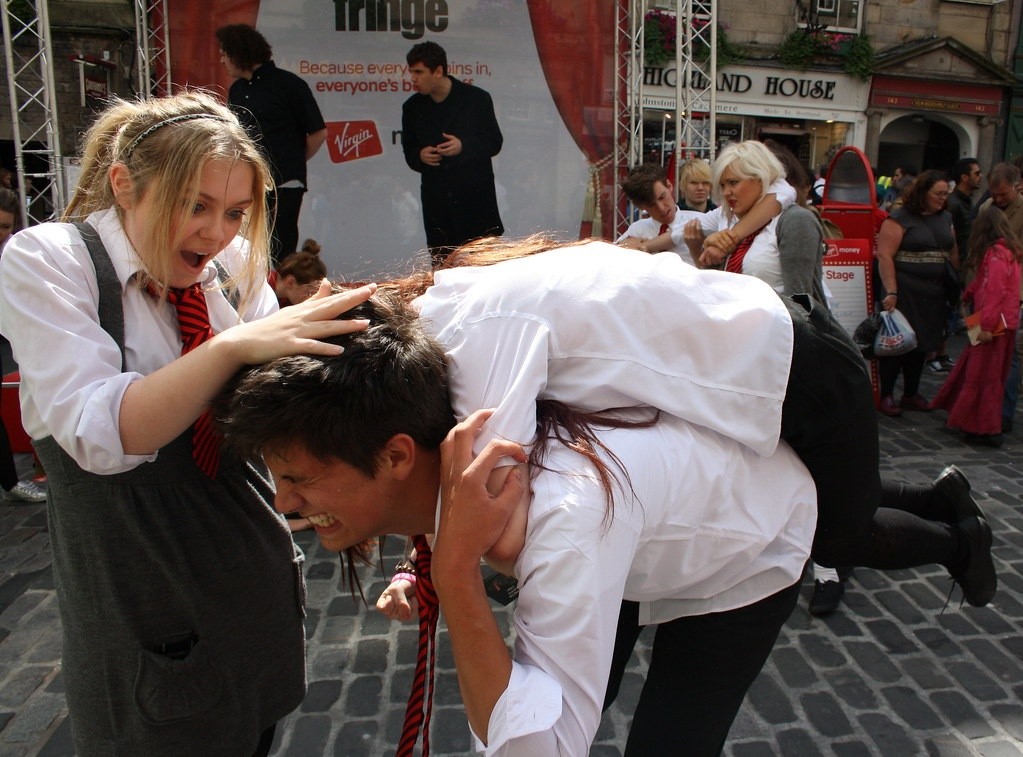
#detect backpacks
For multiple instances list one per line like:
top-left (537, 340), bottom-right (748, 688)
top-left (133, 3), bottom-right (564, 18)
top-left (808, 183), bottom-right (826, 204)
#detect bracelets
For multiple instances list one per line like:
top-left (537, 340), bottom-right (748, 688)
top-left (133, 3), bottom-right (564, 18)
top-left (394, 566), bottom-right (416, 575)
top-left (391, 573), bottom-right (416, 585)
top-left (888, 293), bottom-right (897, 295)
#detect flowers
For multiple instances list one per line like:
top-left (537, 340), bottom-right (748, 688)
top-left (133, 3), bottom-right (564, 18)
top-left (641, 7), bottom-right (730, 65)
top-left (780, 28), bottom-right (873, 83)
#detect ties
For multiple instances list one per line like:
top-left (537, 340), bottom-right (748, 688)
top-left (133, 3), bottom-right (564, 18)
top-left (726, 218), bottom-right (771, 275)
top-left (396, 533), bottom-right (440, 757)
top-left (133, 269), bottom-right (222, 483)
top-left (658, 222), bottom-right (669, 254)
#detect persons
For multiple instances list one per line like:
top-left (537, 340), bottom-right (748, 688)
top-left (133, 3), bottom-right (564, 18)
top-left (816, 158), bottom-right (1023, 449)
top-left (215, 24), bottom-right (328, 265)
top-left (268, 240), bottom-right (327, 304)
top-left (400, 40), bottom-right (505, 264)
top-left (0, 168), bottom-right (48, 504)
top-left (229, 321), bottom-right (818, 757)
top-left (0, 90), bottom-right (377, 757)
top-left (376, 234), bottom-right (997, 622)
top-left (613, 141), bottom-right (828, 307)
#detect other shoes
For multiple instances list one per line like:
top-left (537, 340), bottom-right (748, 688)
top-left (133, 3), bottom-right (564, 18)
top-left (939, 354), bottom-right (955, 368)
top-left (810, 580), bottom-right (844, 615)
top-left (880, 395), bottom-right (903, 418)
top-left (962, 431), bottom-right (1002, 447)
top-left (924, 361), bottom-right (950, 376)
top-left (1001, 419), bottom-right (1013, 432)
top-left (901, 393), bottom-right (935, 411)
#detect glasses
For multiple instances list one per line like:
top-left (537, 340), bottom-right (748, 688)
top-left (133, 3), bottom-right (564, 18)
top-left (965, 169), bottom-right (983, 177)
top-left (929, 191), bottom-right (951, 199)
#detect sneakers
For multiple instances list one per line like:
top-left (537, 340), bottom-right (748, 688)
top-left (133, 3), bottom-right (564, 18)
top-left (947, 516), bottom-right (998, 607)
top-left (932, 464), bottom-right (986, 526)
top-left (1, 484), bottom-right (48, 503)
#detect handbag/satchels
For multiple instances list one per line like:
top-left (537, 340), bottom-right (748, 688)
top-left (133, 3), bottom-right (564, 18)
top-left (873, 307), bottom-right (918, 356)
top-left (943, 257), bottom-right (962, 304)
